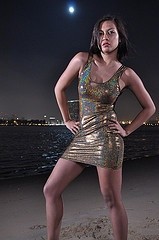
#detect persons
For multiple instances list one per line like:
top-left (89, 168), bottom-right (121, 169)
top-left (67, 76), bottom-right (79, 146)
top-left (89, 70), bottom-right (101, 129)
top-left (43, 16), bottom-right (156, 240)
